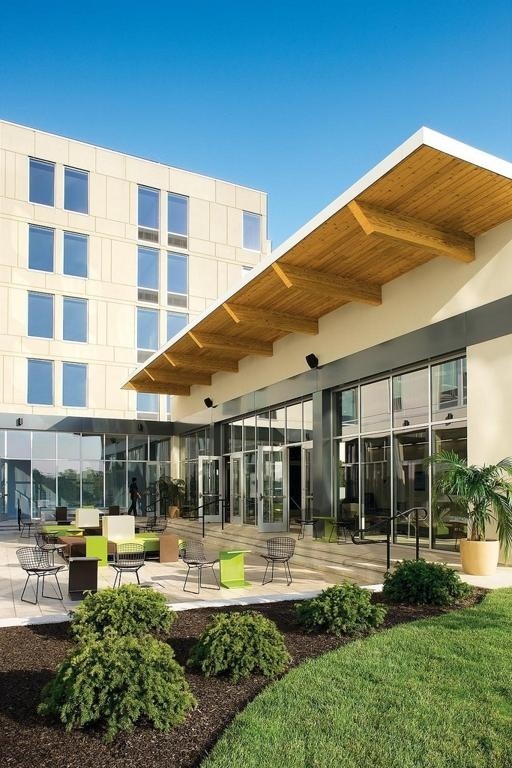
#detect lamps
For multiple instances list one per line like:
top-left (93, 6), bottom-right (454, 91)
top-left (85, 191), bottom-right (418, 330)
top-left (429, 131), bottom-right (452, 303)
top-left (137, 424), bottom-right (146, 432)
top-left (305, 353), bottom-right (324, 371)
top-left (204, 396), bottom-right (218, 408)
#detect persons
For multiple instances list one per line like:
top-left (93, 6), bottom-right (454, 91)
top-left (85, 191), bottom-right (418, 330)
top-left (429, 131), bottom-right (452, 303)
top-left (127, 476), bottom-right (142, 515)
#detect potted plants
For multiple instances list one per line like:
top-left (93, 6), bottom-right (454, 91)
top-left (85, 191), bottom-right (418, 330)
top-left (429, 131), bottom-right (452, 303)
top-left (413, 443), bottom-right (512, 577)
